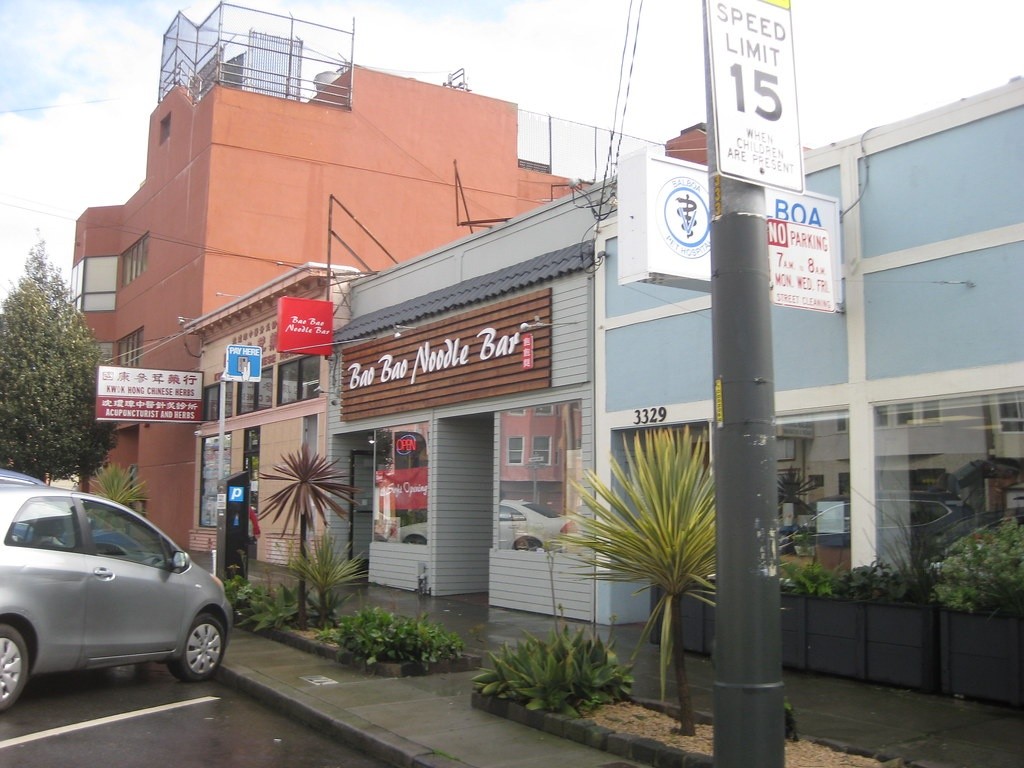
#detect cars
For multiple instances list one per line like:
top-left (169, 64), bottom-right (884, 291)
top-left (394, 497), bottom-right (582, 552)
top-left (0, 467), bottom-right (154, 557)
top-left (1, 478), bottom-right (235, 714)
top-left (831, 506), bottom-right (1024, 586)
top-left (778, 486), bottom-right (975, 554)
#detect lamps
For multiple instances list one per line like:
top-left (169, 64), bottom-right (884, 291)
top-left (217, 293), bottom-right (241, 299)
top-left (277, 263), bottom-right (303, 269)
top-left (178, 317), bottom-right (195, 325)
top-left (368, 433), bottom-right (377, 444)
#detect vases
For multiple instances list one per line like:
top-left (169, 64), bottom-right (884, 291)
top-left (939, 607), bottom-right (1024, 709)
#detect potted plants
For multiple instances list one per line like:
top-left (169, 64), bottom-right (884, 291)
top-left (663, 473), bottom-right (935, 689)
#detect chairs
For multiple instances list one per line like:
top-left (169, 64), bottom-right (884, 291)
top-left (33, 517), bottom-right (64, 547)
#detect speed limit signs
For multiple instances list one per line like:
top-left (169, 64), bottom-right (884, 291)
top-left (705, 0), bottom-right (808, 195)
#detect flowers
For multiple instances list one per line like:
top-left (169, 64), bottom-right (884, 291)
top-left (925, 515), bottom-right (1024, 620)
